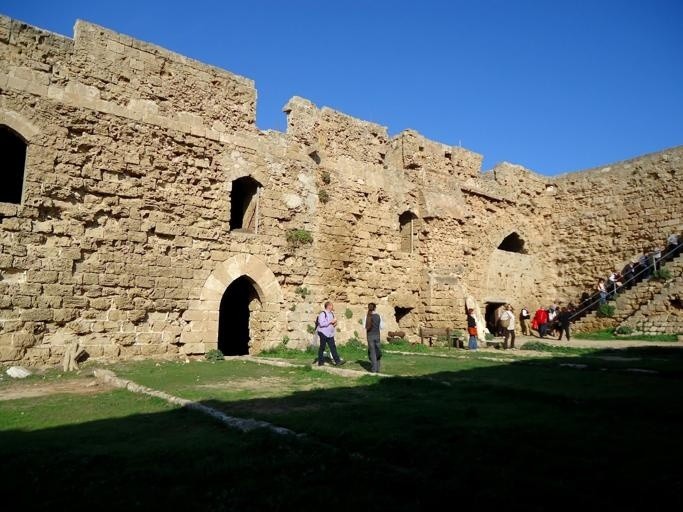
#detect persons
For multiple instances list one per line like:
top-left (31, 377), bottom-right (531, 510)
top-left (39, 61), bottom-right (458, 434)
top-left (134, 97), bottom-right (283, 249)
top-left (495, 302), bottom-right (507, 335)
top-left (466, 308), bottom-right (478, 349)
top-left (520, 304), bottom-right (531, 337)
top-left (596, 229), bottom-right (681, 304)
top-left (312, 301), bottom-right (347, 366)
top-left (534, 288), bottom-right (590, 340)
top-left (364, 302), bottom-right (383, 372)
top-left (497, 303), bottom-right (519, 351)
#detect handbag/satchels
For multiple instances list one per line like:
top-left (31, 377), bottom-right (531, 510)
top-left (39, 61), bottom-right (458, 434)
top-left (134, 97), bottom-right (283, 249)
top-left (499, 311), bottom-right (511, 328)
top-left (468, 327), bottom-right (477, 336)
top-left (368, 345), bottom-right (383, 361)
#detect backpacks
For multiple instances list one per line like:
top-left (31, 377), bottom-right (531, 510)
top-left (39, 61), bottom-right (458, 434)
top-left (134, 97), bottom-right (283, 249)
top-left (315, 311), bottom-right (327, 330)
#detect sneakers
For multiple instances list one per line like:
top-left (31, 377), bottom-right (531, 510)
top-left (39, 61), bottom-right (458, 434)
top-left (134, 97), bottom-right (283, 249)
top-left (335, 360), bottom-right (345, 367)
